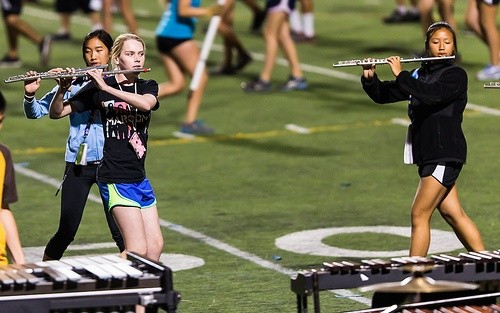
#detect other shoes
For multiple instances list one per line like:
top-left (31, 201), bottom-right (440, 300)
top-left (477, 65), bottom-right (500, 81)
top-left (213, 66), bottom-right (232, 75)
top-left (242, 78), bottom-right (270, 93)
top-left (384, 9), bottom-right (406, 23)
top-left (279, 75), bottom-right (308, 91)
top-left (237, 54), bottom-right (251, 70)
top-left (401, 9), bottom-right (420, 22)
top-left (54, 33), bottom-right (70, 40)
top-left (0, 55), bottom-right (22, 69)
top-left (174, 122), bottom-right (214, 141)
top-left (39, 35), bottom-right (52, 65)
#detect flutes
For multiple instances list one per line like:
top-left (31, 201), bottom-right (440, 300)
top-left (40, 68), bottom-right (152, 80)
top-left (331, 56), bottom-right (456, 67)
top-left (5, 63), bottom-right (109, 83)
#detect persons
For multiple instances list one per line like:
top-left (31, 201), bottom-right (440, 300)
top-left (144, 0), bottom-right (226, 136)
top-left (0, 0), bottom-right (51, 67)
top-left (383, 0), bottom-right (462, 63)
top-left (0, 89), bottom-right (27, 266)
top-left (288, 0), bottom-right (316, 42)
top-left (23, 29), bottom-right (125, 262)
top-left (361, 22), bottom-right (486, 256)
top-left (50, 0), bottom-right (100, 41)
top-left (243, 0), bottom-right (310, 93)
top-left (465, 0), bottom-right (500, 81)
top-left (101, 0), bottom-right (138, 36)
top-left (49, 33), bottom-right (164, 313)
top-left (207, 0), bottom-right (252, 75)
